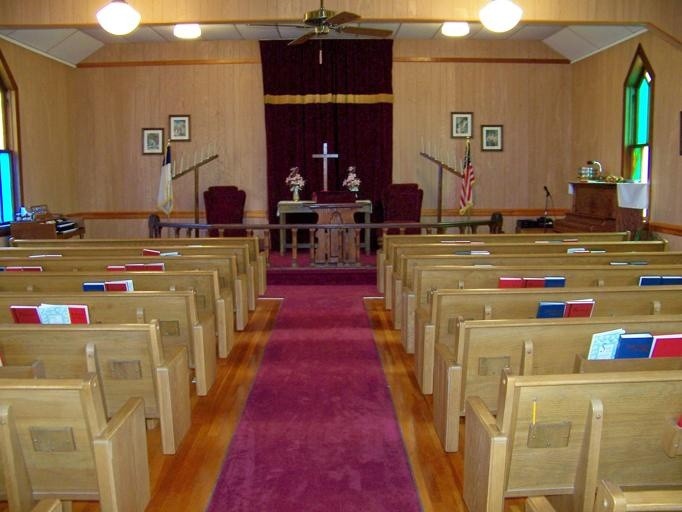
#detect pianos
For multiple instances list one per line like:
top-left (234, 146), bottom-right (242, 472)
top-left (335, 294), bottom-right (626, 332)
top-left (552, 181), bottom-right (642, 233)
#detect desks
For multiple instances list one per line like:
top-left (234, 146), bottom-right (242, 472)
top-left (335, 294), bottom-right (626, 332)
top-left (276, 200), bottom-right (372, 258)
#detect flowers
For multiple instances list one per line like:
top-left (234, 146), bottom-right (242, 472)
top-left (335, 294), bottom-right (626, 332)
top-left (284, 164), bottom-right (305, 191)
top-left (342, 165), bottom-right (361, 192)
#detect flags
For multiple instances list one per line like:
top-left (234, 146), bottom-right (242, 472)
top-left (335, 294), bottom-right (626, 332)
top-left (156, 144), bottom-right (176, 217)
top-left (456, 141), bottom-right (475, 215)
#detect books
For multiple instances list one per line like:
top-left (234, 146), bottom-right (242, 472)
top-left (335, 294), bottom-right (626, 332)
top-left (536, 301), bottom-right (565, 319)
top-left (0, 266), bottom-right (43, 271)
top-left (499, 276), bottom-right (566, 288)
top-left (608, 261), bottom-right (645, 265)
top-left (8, 304), bottom-right (41, 324)
top-left (586, 328), bottom-right (625, 361)
top-left (647, 334), bottom-right (682, 359)
top-left (39, 304), bottom-right (90, 325)
top-left (662, 276), bottom-right (682, 286)
top-left (573, 250), bottom-right (607, 254)
top-left (614, 332), bottom-right (653, 360)
top-left (106, 261), bottom-right (165, 271)
top-left (82, 280), bottom-right (134, 293)
top-left (456, 250), bottom-right (491, 255)
top-left (564, 300), bottom-right (595, 319)
top-left (639, 276), bottom-right (662, 286)
top-left (141, 248), bottom-right (179, 257)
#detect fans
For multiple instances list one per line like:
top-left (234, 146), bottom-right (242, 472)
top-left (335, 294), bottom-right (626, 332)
top-left (236, 0), bottom-right (393, 46)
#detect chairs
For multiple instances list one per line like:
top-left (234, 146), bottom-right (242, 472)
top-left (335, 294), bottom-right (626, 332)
top-left (377, 183), bottom-right (424, 248)
top-left (204, 186), bottom-right (247, 238)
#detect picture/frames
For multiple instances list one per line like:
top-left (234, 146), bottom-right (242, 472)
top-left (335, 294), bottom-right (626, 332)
top-left (481, 124), bottom-right (503, 152)
top-left (451, 112), bottom-right (472, 140)
top-left (167, 114), bottom-right (191, 142)
top-left (142, 127), bottom-right (163, 155)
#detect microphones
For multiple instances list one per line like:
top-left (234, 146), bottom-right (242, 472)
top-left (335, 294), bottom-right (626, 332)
top-left (542, 185), bottom-right (551, 197)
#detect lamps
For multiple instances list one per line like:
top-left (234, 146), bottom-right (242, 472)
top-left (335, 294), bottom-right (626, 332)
top-left (98, 0), bottom-right (142, 35)
top-left (440, 21), bottom-right (471, 38)
top-left (174, 23), bottom-right (201, 40)
top-left (478, 0), bottom-right (525, 32)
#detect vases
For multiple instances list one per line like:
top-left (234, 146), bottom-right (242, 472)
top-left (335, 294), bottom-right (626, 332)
top-left (291, 192), bottom-right (301, 201)
